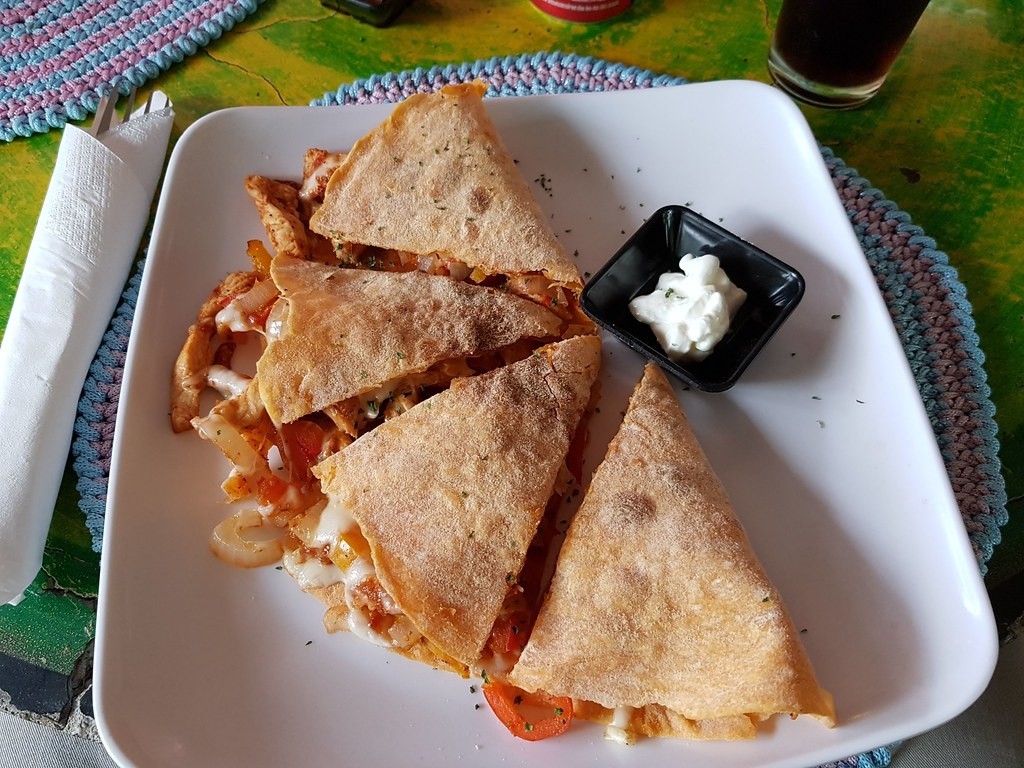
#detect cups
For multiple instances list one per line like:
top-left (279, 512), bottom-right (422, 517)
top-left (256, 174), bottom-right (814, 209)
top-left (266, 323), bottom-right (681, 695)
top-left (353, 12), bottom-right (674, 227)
top-left (766, 0), bottom-right (933, 110)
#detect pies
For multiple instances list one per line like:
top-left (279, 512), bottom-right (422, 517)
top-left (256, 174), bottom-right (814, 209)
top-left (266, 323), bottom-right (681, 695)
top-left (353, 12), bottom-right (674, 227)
top-left (167, 80), bottom-right (838, 745)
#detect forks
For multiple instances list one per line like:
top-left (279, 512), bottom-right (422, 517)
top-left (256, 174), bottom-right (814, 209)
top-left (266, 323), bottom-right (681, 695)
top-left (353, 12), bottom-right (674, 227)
top-left (96, 81), bottom-right (171, 139)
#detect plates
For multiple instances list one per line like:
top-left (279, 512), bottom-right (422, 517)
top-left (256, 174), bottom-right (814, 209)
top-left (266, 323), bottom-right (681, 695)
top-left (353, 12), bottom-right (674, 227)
top-left (93, 79), bottom-right (999, 768)
top-left (583, 207), bottom-right (806, 393)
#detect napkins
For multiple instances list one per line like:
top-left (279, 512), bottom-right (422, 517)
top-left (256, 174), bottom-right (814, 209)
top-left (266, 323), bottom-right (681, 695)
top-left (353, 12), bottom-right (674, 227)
top-left (0, 87), bottom-right (176, 609)
